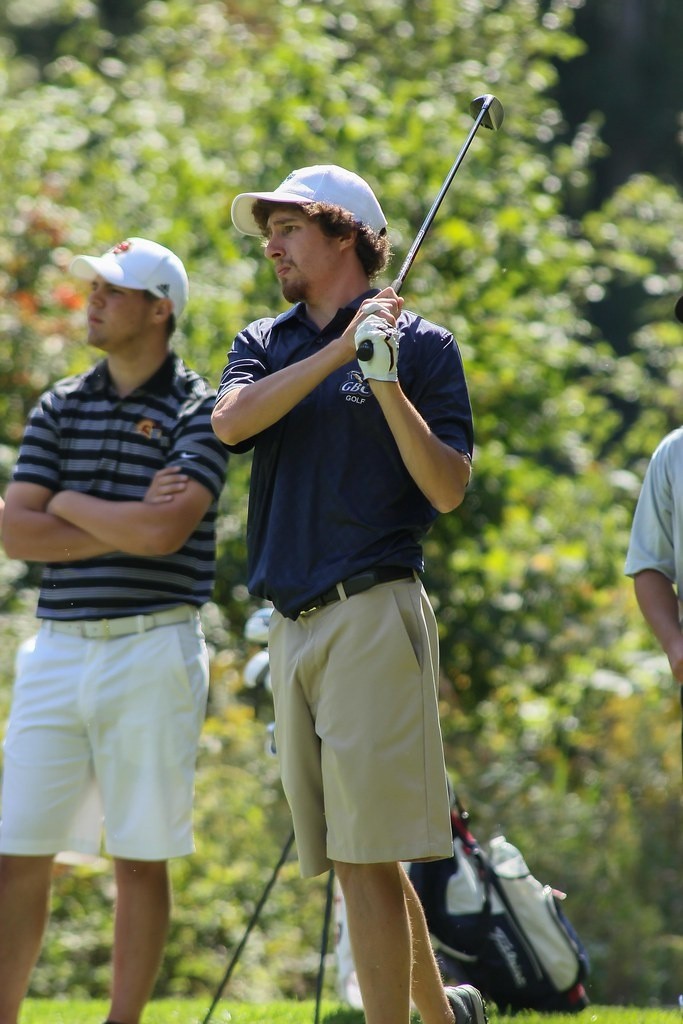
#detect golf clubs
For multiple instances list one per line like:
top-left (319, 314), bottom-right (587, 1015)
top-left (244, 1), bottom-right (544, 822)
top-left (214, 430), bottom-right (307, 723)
top-left (355, 94), bottom-right (504, 362)
top-left (241, 607), bottom-right (277, 756)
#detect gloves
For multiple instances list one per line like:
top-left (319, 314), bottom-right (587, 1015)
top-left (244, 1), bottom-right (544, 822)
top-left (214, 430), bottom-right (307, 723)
top-left (355, 314), bottom-right (398, 382)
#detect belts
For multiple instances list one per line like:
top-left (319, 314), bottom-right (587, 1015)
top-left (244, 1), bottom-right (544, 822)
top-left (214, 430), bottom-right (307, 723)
top-left (39, 603), bottom-right (198, 642)
top-left (297, 565), bottom-right (413, 616)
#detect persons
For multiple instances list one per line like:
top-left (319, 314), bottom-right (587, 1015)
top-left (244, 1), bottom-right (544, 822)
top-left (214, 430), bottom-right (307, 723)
top-left (623, 424), bottom-right (683, 691)
top-left (210, 163), bottom-right (474, 1024)
top-left (0, 234), bottom-right (228, 1024)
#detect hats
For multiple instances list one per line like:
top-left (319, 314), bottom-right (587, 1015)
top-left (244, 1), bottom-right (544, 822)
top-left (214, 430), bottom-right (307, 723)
top-left (70, 236), bottom-right (187, 319)
top-left (230, 164), bottom-right (387, 238)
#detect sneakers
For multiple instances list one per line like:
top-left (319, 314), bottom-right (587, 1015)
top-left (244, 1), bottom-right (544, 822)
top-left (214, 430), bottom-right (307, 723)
top-left (443, 984), bottom-right (489, 1024)
